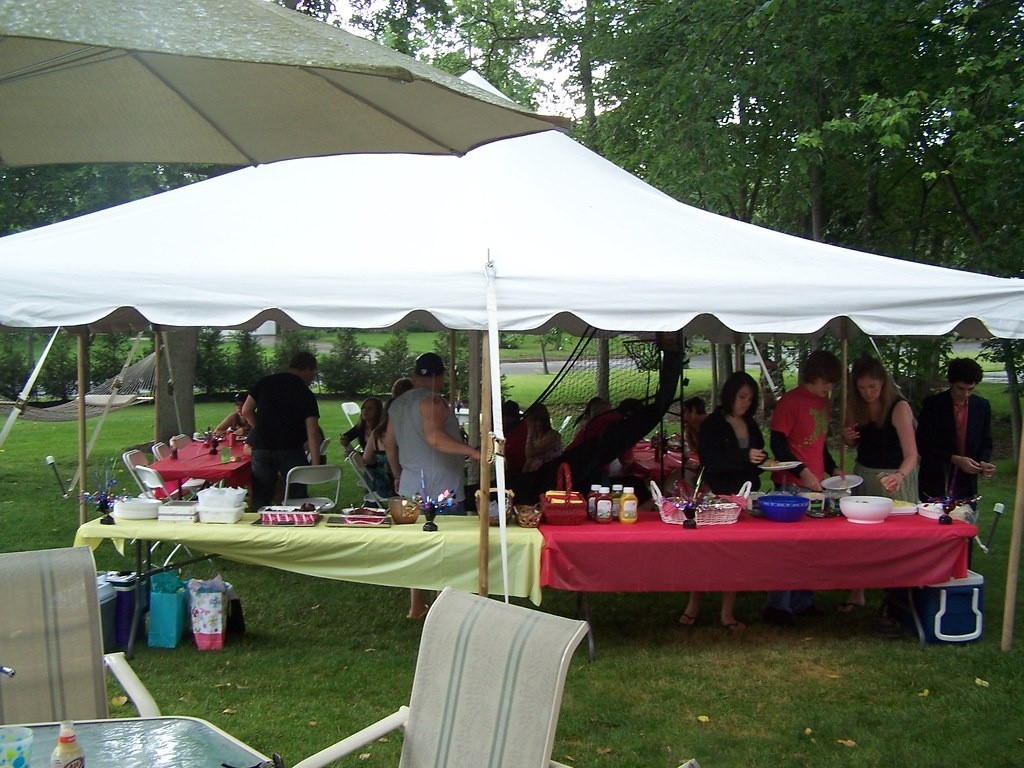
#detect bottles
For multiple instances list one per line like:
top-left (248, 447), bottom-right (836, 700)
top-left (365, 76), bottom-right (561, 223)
top-left (684, 442), bottom-right (690, 463)
top-left (595, 487), bottom-right (613, 523)
top-left (586, 485), bottom-right (602, 520)
top-left (226, 426), bottom-right (233, 445)
top-left (170, 436), bottom-right (178, 459)
top-left (207, 426), bottom-right (212, 438)
top-left (619, 486), bottom-right (637, 523)
top-left (51, 720), bottom-right (85, 768)
top-left (194, 437), bottom-right (222, 442)
top-left (610, 484), bottom-right (623, 520)
top-left (650, 428), bottom-right (665, 462)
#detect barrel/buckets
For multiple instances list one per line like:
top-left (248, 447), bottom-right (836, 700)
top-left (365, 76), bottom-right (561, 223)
top-left (95, 570), bottom-right (148, 653)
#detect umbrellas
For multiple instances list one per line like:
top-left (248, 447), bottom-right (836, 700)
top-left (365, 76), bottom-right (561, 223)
top-left (0, 3), bottom-right (571, 168)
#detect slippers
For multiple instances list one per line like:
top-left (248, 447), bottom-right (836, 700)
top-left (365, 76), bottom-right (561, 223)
top-left (406, 604), bottom-right (429, 618)
top-left (725, 620), bottom-right (746, 631)
top-left (835, 602), bottom-right (867, 615)
top-left (679, 613), bottom-right (698, 626)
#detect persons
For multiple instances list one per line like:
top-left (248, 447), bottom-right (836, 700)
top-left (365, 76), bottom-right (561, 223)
top-left (384, 352), bottom-right (481, 620)
top-left (212, 391), bottom-right (255, 435)
top-left (684, 396), bottom-right (709, 454)
top-left (770, 350), bottom-right (852, 619)
top-left (503, 400), bottom-right (563, 507)
top-left (339, 397), bottom-right (384, 452)
top-left (834, 355), bottom-right (921, 614)
top-left (915, 357), bottom-right (997, 572)
top-left (571, 397), bottom-right (643, 470)
top-left (362, 378), bottom-right (417, 499)
top-left (242, 350), bottom-right (327, 513)
top-left (677, 369), bottom-right (768, 635)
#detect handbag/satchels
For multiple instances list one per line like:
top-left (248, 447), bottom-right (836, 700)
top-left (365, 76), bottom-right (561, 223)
top-left (188, 578), bottom-right (232, 649)
top-left (148, 583), bottom-right (188, 648)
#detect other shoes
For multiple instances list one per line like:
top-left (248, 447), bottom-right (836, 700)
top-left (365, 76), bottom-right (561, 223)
top-left (796, 604), bottom-right (825, 617)
top-left (763, 605), bottom-right (797, 629)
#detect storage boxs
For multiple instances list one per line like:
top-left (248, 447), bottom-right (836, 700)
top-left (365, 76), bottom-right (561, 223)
top-left (96, 571), bottom-right (146, 653)
top-left (257, 505), bottom-right (322, 524)
top-left (196, 501), bottom-right (248, 524)
top-left (196, 487), bottom-right (248, 507)
top-left (886, 568), bottom-right (986, 645)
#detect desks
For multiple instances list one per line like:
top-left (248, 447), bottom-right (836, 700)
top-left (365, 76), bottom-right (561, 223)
top-left (0, 716), bottom-right (280, 768)
top-left (539, 512), bottom-right (978, 662)
top-left (72, 510), bottom-right (546, 661)
top-left (147, 430), bottom-right (253, 500)
top-left (618, 433), bottom-right (701, 501)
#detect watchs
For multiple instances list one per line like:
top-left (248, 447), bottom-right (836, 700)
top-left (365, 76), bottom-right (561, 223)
top-left (895, 470), bottom-right (907, 480)
top-left (393, 469), bottom-right (404, 481)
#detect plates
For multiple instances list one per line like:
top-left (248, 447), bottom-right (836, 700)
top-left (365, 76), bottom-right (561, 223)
top-left (113, 498), bottom-right (162, 519)
top-left (757, 461), bottom-right (802, 470)
top-left (235, 435), bottom-right (248, 440)
top-left (821, 474), bottom-right (863, 489)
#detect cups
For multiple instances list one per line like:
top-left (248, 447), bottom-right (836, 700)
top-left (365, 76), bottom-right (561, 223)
top-left (0, 727), bottom-right (33, 768)
top-left (221, 447), bottom-right (231, 463)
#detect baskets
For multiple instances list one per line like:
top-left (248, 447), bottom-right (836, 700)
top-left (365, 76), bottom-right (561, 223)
top-left (514, 505), bottom-right (542, 527)
top-left (650, 480), bottom-right (753, 525)
top-left (475, 488), bottom-right (514, 526)
top-left (541, 463), bottom-right (587, 524)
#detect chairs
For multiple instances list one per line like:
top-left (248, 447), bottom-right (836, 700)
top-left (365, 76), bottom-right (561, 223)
top-left (290, 585), bottom-right (591, 768)
top-left (123, 402), bottom-right (406, 573)
top-left (0, 544), bottom-right (162, 729)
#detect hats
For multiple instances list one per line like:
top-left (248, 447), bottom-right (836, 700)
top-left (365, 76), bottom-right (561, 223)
top-left (414, 352), bottom-right (447, 374)
top-left (230, 393), bottom-right (247, 402)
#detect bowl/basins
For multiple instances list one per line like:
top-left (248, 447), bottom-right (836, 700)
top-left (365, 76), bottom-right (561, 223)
top-left (917, 503), bottom-right (966, 519)
top-left (889, 503), bottom-right (918, 515)
top-left (388, 496), bottom-right (420, 524)
top-left (757, 494), bottom-right (810, 520)
top-left (342, 507), bottom-right (387, 524)
top-left (197, 488), bottom-right (248, 507)
top-left (839, 496), bottom-right (893, 523)
top-left (195, 502), bottom-right (247, 524)
top-left (259, 505), bottom-right (321, 523)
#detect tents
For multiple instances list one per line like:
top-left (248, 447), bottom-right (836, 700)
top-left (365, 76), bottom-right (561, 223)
top-left (0, 64), bottom-right (1024, 654)
